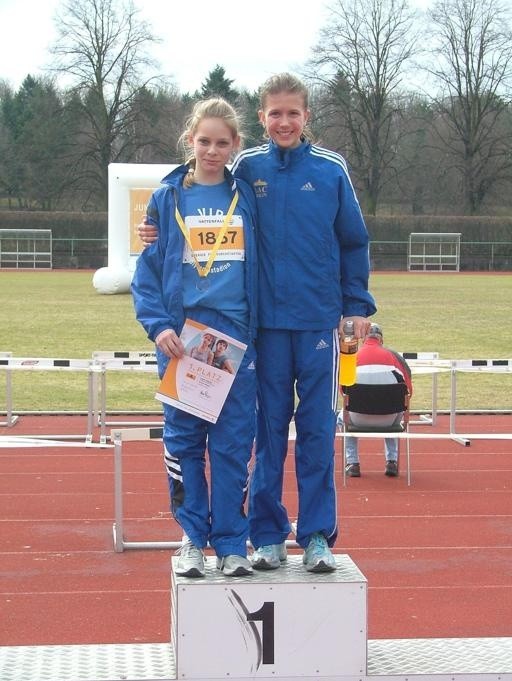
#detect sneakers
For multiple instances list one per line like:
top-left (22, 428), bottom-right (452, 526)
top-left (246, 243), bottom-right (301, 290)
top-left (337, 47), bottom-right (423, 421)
top-left (384, 462), bottom-right (397, 476)
top-left (346, 464), bottom-right (361, 477)
top-left (217, 555), bottom-right (253, 577)
top-left (250, 541), bottom-right (287, 569)
top-left (303, 535), bottom-right (337, 573)
top-left (174, 539), bottom-right (207, 578)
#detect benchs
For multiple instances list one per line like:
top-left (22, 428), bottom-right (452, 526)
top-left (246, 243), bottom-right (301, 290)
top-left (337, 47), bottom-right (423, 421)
top-left (0, 251), bottom-right (52, 270)
top-left (406, 254), bottom-right (460, 272)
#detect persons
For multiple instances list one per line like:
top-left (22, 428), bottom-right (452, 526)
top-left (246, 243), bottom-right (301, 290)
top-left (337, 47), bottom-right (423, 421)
top-left (190, 333), bottom-right (217, 366)
top-left (211, 340), bottom-right (234, 374)
top-left (337, 323), bottom-right (413, 477)
top-left (129, 98), bottom-right (259, 575)
top-left (138, 72), bottom-right (377, 572)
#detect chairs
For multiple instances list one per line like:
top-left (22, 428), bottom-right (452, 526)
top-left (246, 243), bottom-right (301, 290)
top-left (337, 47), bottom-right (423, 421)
top-left (340, 382), bottom-right (411, 487)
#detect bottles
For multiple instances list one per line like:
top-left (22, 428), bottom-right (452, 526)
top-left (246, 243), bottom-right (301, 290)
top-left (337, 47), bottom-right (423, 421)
top-left (338, 318), bottom-right (358, 388)
